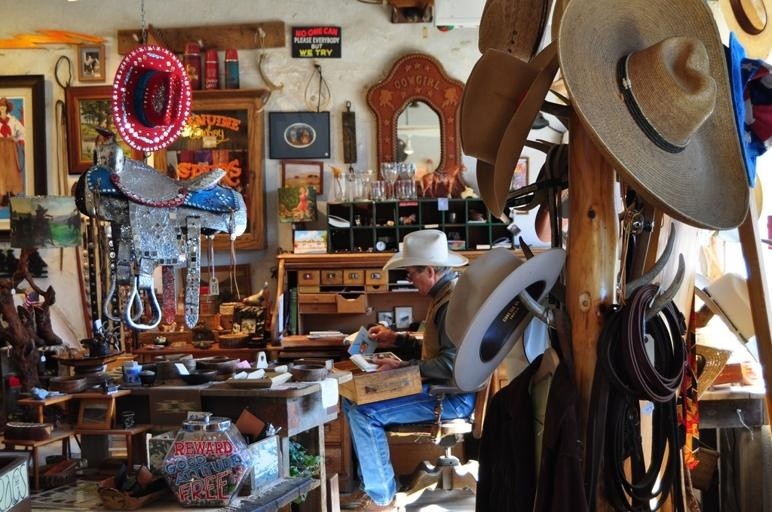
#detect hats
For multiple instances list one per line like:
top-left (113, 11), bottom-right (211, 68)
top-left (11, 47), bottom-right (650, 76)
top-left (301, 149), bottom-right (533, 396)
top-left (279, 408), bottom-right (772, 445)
top-left (692, 272), bottom-right (761, 367)
top-left (382, 228), bottom-right (470, 270)
top-left (459, 39), bottom-right (561, 220)
top-left (560, 0), bottom-right (750, 230)
top-left (444, 249), bottom-right (568, 394)
top-left (730, 30), bottom-right (770, 188)
top-left (110, 44), bottom-right (192, 153)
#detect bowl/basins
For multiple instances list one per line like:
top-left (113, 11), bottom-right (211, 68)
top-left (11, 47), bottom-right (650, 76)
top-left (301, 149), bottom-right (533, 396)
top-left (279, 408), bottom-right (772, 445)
top-left (219, 332), bottom-right (248, 347)
top-left (48, 375), bottom-right (87, 391)
top-left (154, 354), bottom-right (327, 385)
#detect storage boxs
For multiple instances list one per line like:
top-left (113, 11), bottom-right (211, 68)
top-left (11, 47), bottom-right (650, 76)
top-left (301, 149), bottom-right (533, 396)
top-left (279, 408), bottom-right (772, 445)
top-left (333, 359), bottom-right (423, 406)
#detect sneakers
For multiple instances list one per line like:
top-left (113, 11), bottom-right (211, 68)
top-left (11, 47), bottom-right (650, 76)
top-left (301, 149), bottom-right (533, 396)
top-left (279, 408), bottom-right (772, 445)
top-left (340, 488), bottom-right (398, 510)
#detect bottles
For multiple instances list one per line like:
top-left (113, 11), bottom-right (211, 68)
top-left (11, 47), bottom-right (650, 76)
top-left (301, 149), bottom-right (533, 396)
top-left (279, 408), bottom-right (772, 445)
top-left (124, 364), bottom-right (142, 387)
top-left (153, 337), bottom-right (169, 346)
top-left (342, 330), bottom-right (361, 346)
top-left (159, 417), bottom-right (258, 506)
top-left (184, 43), bottom-right (239, 90)
top-left (712, 360), bottom-right (753, 383)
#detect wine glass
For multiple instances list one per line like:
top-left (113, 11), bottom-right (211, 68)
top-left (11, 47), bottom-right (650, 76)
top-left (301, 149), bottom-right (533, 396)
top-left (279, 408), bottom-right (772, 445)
top-left (137, 373), bottom-right (155, 387)
top-left (330, 163), bottom-right (416, 203)
top-left (53, 348), bottom-right (125, 383)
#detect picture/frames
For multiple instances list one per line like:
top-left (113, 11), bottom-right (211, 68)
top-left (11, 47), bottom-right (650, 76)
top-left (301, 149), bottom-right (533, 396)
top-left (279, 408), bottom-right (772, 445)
top-left (0, 75), bottom-right (46, 242)
top-left (510, 157), bottom-right (529, 215)
top-left (181, 264), bottom-right (252, 327)
top-left (76, 44), bottom-right (106, 82)
top-left (76, 398), bottom-right (116, 430)
top-left (269, 112), bottom-right (330, 159)
top-left (292, 229), bottom-right (328, 254)
top-left (282, 160), bottom-right (323, 195)
top-left (64, 86), bottom-right (145, 175)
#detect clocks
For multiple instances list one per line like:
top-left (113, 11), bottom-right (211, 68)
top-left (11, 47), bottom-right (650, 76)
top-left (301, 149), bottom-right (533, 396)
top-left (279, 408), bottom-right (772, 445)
top-left (730, 0), bottom-right (767, 35)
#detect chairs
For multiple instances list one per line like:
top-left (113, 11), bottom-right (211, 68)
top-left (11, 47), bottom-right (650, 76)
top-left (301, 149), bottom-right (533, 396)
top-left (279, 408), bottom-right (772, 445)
top-left (383, 361), bottom-right (508, 512)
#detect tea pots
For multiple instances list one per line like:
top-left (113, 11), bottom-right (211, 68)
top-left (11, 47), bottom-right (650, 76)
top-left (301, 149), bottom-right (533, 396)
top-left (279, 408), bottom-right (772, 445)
top-left (81, 332), bottom-right (117, 357)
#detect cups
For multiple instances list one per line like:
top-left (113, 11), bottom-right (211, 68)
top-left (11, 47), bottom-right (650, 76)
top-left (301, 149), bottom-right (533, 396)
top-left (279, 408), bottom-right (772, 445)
top-left (449, 211), bottom-right (459, 223)
top-left (123, 411), bottom-right (137, 428)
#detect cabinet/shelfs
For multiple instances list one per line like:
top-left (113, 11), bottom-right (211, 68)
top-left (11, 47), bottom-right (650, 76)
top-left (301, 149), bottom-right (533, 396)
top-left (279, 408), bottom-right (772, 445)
top-left (323, 412), bottom-right (356, 494)
top-left (325, 199), bottom-right (516, 254)
top-left (0, 388), bottom-right (153, 493)
top-left (153, 88), bottom-right (268, 251)
top-left (274, 248), bottom-right (553, 358)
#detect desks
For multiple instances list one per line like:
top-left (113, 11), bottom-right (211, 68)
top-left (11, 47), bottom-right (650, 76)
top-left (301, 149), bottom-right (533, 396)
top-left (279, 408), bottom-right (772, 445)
top-left (90, 370), bottom-right (353, 512)
top-left (132, 341), bottom-right (273, 367)
top-left (697, 375), bottom-right (764, 512)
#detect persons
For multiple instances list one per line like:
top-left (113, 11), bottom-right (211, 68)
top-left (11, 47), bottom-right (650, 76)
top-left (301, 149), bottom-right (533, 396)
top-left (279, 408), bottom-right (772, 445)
top-left (294, 186), bottom-right (314, 220)
top-left (339, 230), bottom-right (476, 512)
top-left (0, 98), bottom-right (25, 207)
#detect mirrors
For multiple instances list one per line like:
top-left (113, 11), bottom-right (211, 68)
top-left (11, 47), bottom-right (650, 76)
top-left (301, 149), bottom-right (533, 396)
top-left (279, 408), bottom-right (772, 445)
top-left (368, 53), bottom-right (467, 199)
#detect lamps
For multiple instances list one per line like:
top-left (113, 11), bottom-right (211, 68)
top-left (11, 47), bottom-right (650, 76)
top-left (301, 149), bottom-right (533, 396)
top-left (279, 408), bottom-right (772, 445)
top-left (410, 101), bottom-right (418, 109)
top-left (404, 106), bottom-right (414, 155)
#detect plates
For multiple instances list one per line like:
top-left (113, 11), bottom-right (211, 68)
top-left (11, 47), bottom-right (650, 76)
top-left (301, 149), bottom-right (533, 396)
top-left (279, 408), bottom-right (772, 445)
top-left (192, 340), bottom-right (213, 348)
